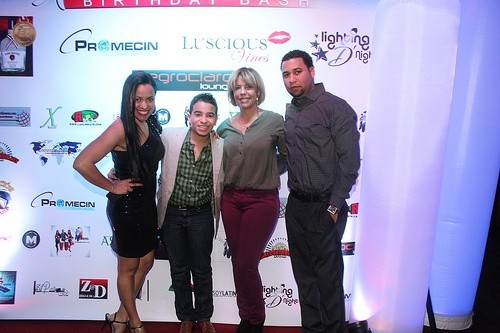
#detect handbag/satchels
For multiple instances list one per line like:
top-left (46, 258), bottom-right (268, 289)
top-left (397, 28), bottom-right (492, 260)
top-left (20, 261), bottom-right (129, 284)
top-left (155, 228), bottom-right (169, 260)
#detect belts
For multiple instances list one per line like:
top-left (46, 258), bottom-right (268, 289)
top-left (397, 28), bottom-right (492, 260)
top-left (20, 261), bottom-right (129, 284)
top-left (289, 189), bottom-right (330, 202)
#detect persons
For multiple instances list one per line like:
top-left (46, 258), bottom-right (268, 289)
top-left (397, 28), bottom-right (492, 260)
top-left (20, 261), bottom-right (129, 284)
top-left (72, 71), bottom-right (219, 333)
top-left (217, 67), bottom-right (288, 333)
top-left (275, 50), bottom-right (361, 333)
top-left (106, 93), bottom-right (224, 333)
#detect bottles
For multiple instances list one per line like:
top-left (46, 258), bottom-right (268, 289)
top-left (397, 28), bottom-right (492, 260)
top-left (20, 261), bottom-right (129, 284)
top-left (0, 17), bottom-right (26, 73)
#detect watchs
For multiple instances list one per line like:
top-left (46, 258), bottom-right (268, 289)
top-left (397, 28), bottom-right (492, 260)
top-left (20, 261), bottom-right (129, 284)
top-left (327, 206), bottom-right (339, 215)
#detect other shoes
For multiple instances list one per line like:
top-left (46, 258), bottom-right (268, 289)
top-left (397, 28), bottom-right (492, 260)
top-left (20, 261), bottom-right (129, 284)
top-left (236, 320), bottom-right (250, 333)
top-left (248, 324), bottom-right (262, 333)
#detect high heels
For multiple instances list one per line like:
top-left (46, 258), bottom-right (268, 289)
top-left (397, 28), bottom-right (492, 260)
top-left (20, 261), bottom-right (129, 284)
top-left (101, 312), bottom-right (129, 333)
top-left (127, 320), bottom-right (146, 333)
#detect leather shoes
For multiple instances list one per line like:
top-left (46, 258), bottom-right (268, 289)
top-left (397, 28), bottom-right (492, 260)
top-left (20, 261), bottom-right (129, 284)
top-left (180, 320), bottom-right (196, 333)
top-left (199, 320), bottom-right (215, 333)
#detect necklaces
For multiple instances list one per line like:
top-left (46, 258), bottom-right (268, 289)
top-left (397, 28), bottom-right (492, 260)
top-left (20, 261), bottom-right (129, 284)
top-left (237, 117), bottom-right (245, 127)
